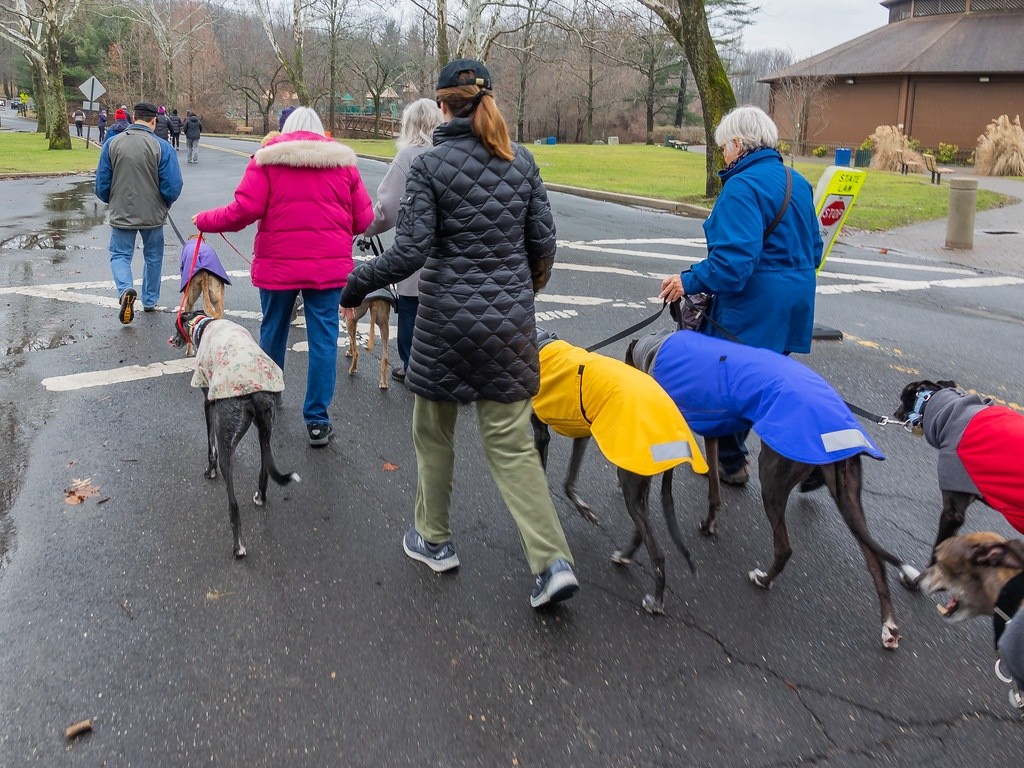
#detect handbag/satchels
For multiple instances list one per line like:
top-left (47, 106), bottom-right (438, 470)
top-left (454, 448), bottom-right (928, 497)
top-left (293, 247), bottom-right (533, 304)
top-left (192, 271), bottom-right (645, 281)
top-left (669, 292), bottom-right (710, 334)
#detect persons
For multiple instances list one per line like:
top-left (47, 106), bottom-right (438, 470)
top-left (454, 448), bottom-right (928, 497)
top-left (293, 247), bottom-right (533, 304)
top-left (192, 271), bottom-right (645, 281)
top-left (154, 105), bottom-right (174, 141)
top-left (120, 104), bottom-right (132, 124)
top-left (72, 108), bottom-right (86, 136)
top-left (659, 104), bottom-right (824, 484)
top-left (339, 55), bottom-right (582, 606)
top-left (170, 108), bottom-right (182, 150)
top-left (365, 97), bottom-right (444, 382)
top-left (183, 109), bottom-right (194, 150)
top-left (94, 102), bottom-right (184, 324)
top-left (261, 106), bottom-right (295, 148)
top-left (184, 111), bottom-right (202, 163)
top-left (190, 104), bottom-right (376, 447)
top-left (97, 110), bottom-right (108, 141)
top-left (102, 108), bottom-right (131, 144)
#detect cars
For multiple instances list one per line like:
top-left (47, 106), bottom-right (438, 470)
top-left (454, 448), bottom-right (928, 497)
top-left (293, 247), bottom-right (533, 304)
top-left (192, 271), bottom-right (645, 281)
top-left (11, 98), bottom-right (22, 110)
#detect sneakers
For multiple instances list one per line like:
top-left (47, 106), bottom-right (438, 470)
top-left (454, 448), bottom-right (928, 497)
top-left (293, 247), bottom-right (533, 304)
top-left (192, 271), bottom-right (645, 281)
top-left (402, 529), bottom-right (460, 571)
top-left (308, 422), bottom-right (333, 444)
top-left (530, 558), bottom-right (579, 607)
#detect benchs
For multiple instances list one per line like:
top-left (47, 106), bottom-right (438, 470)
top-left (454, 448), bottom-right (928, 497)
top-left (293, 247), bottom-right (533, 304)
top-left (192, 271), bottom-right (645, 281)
top-left (924, 154), bottom-right (954, 186)
top-left (669, 140), bottom-right (689, 150)
top-left (896, 149), bottom-right (917, 175)
top-left (234, 127), bottom-right (253, 134)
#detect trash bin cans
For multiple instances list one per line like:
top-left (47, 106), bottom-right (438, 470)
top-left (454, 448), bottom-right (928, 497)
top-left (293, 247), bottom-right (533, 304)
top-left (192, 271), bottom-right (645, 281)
top-left (835, 148), bottom-right (851, 166)
top-left (542, 138), bottom-right (547, 144)
top-left (854, 150), bottom-right (871, 167)
top-left (548, 137), bottom-right (556, 144)
top-left (665, 135), bottom-right (674, 147)
top-left (325, 131), bottom-right (331, 137)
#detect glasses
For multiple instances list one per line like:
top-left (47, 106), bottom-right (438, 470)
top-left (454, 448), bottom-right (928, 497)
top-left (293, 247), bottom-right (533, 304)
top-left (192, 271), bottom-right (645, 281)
top-left (719, 140), bottom-right (731, 153)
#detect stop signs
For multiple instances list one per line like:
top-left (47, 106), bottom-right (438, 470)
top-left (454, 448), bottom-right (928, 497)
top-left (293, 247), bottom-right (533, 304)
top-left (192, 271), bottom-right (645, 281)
top-left (821, 200), bottom-right (845, 227)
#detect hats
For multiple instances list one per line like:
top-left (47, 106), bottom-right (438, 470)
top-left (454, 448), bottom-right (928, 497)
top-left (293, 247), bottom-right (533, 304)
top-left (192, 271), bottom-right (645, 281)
top-left (115, 109), bottom-right (126, 119)
top-left (160, 106), bottom-right (165, 113)
top-left (437, 59), bottom-right (493, 90)
top-left (173, 109), bottom-right (177, 114)
top-left (134, 103), bottom-right (159, 124)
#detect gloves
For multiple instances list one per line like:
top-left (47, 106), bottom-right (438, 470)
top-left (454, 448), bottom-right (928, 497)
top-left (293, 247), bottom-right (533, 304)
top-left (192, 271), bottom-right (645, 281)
top-left (341, 285), bottom-right (365, 309)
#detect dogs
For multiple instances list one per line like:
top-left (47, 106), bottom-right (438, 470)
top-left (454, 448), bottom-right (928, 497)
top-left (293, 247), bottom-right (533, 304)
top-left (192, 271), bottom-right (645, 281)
top-left (171, 308), bottom-right (303, 561)
top-left (531, 326), bottom-right (694, 614)
top-left (182, 231), bottom-right (225, 319)
top-left (624, 328), bottom-right (920, 650)
top-left (893, 377), bottom-right (1024, 591)
top-left (343, 254), bottom-right (392, 391)
top-left (920, 531), bottom-right (1024, 721)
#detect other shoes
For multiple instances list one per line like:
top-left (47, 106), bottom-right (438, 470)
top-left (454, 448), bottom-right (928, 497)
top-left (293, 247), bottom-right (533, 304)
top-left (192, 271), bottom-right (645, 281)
top-left (718, 459), bottom-right (750, 484)
top-left (118, 289), bottom-right (137, 324)
top-left (392, 367), bottom-right (406, 381)
top-left (144, 306), bottom-right (155, 311)
